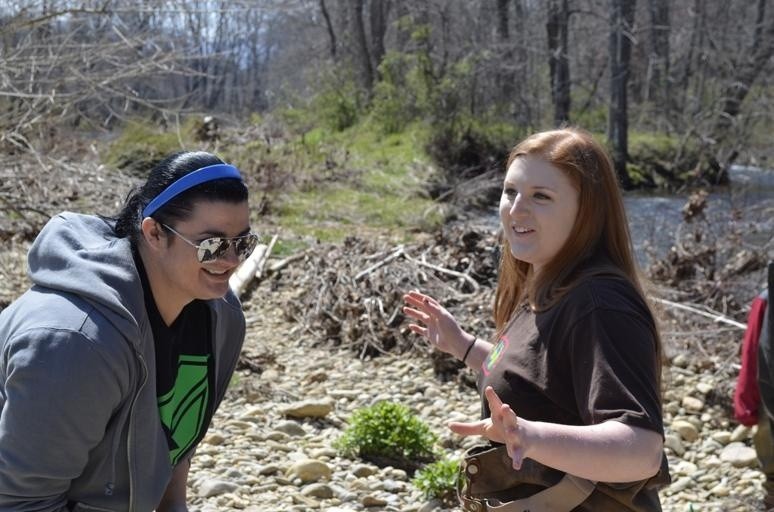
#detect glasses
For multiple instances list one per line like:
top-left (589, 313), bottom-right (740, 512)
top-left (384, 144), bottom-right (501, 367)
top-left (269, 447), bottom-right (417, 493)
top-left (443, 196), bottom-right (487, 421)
top-left (164, 225), bottom-right (258, 264)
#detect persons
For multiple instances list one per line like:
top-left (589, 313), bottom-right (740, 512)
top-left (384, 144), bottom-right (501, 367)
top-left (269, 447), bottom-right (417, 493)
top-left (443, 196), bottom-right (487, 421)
top-left (735, 267), bottom-right (774, 512)
top-left (0, 151), bottom-right (259, 511)
top-left (403, 131), bottom-right (671, 512)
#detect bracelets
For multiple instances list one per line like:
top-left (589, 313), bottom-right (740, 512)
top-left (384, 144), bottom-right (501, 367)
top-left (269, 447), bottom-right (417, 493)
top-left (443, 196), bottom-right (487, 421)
top-left (462, 338), bottom-right (476, 364)
